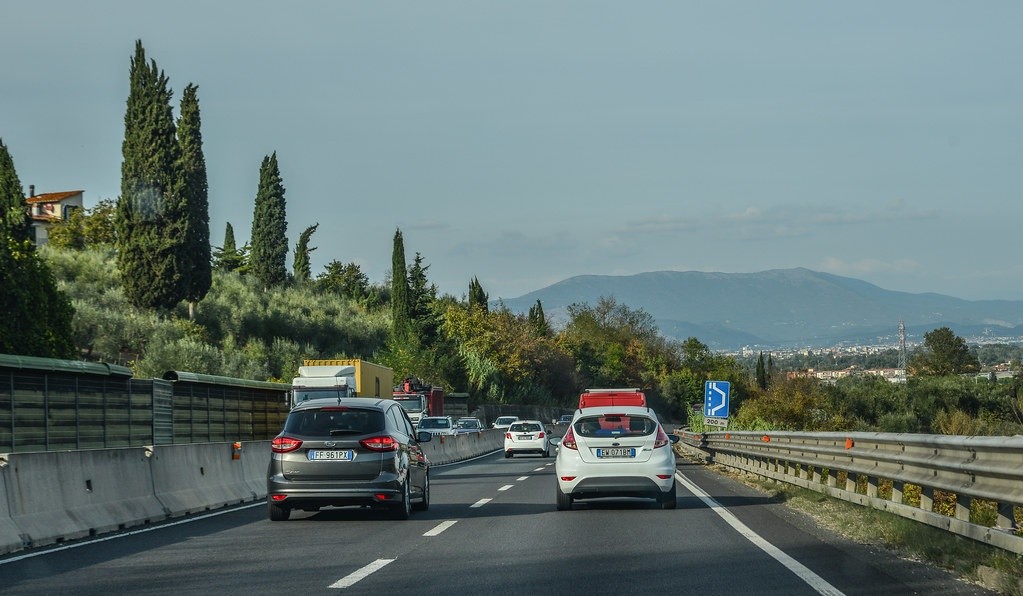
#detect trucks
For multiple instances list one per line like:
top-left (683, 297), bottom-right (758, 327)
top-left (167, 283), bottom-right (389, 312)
top-left (285, 359), bottom-right (393, 412)
top-left (393, 379), bottom-right (444, 431)
top-left (579, 388), bottom-right (647, 408)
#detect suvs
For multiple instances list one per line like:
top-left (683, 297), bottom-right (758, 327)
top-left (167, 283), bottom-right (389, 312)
top-left (266, 374), bottom-right (431, 520)
top-left (504, 419), bottom-right (549, 458)
top-left (553, 406), bottom-right (677, 509)
top-left (415, 416), bottom-right (457, 438)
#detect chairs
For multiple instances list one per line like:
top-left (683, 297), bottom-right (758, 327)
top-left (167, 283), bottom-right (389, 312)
top-left (629, 418), bottom-right (645, 433)
top-left (583, 419), bottom-right (601, 435)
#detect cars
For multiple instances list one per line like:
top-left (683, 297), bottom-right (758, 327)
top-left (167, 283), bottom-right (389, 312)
top-left (454, 417), bottom-right (481, 434)
top-left (492, 416), bottom-right (518, 428)
